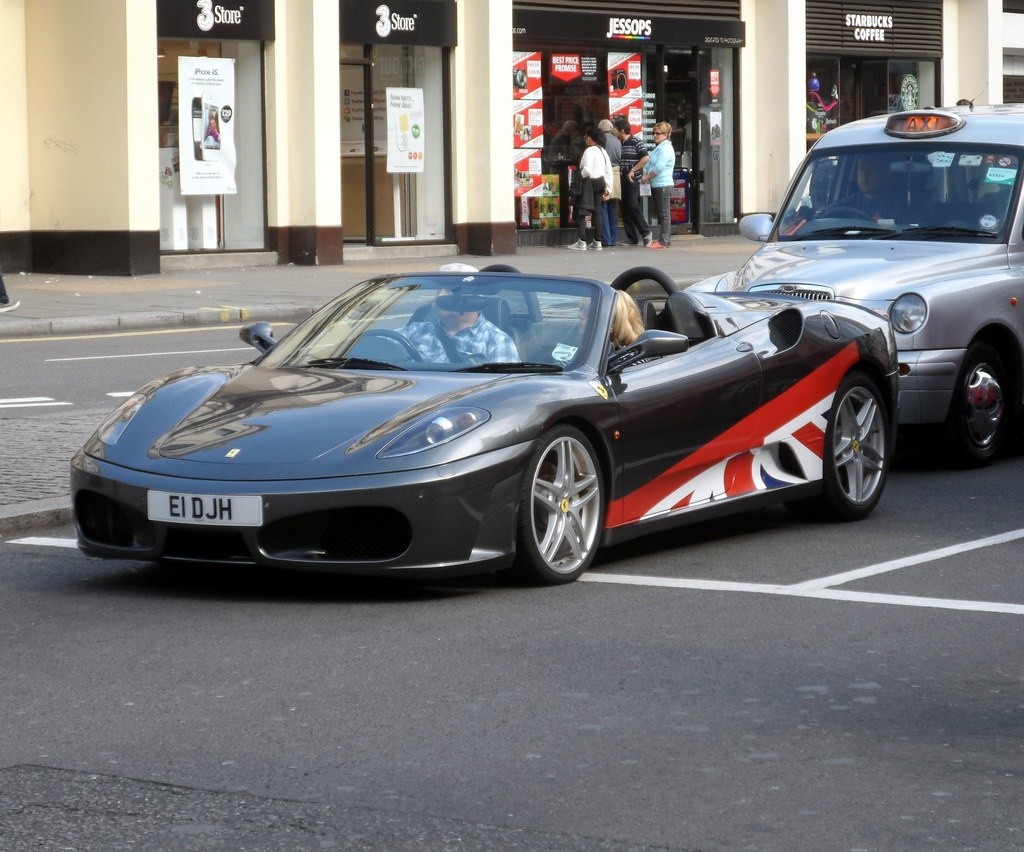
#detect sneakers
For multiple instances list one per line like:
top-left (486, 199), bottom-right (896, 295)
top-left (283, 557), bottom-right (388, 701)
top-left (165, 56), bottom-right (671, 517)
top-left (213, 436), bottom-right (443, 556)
top-left (567, 239), bottom-right (587, 250)
top-left (587, 239), bottom-right (602, 250)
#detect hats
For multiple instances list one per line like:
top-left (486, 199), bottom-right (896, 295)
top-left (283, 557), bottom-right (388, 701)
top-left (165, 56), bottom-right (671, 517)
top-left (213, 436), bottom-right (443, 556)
top-left (439, 263), bottom-right (479, 277)
top-left (597, 119), bottom-right (614, 132)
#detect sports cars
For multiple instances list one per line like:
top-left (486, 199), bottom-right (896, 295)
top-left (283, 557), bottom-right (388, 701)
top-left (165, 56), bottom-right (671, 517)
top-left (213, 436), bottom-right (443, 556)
top-left (69, 264), bottom-right (902, 589)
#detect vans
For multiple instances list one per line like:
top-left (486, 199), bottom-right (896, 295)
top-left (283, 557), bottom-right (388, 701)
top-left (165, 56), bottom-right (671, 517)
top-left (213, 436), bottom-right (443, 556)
top-left (682, 102), bottom-right (1024, 471)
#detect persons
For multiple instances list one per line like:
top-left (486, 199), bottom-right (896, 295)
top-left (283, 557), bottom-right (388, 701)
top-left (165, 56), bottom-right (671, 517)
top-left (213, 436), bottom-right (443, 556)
top-left (567, 128), bottom-right (614, 250)
top-left (571, 289), bottom-right (648, 356)
top-left (642, 121), bottom-right (675, 249)
top-left (812, 146), bottom-right (915, 221)
top-left (378, 263), bottom-right (524, 368)
top-left (596, 118), bottom-right (653, 248)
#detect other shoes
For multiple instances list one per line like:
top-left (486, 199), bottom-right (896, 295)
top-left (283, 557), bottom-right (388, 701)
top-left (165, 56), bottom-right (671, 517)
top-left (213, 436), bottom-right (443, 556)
top-left (619, 240), bottom-right (640, 246)
top-left (643, 230), bottom-right (652, 247)
top-left (602, 244), bottom-right (617, 248)
top-left (647, 240), bottom-right (672, 249)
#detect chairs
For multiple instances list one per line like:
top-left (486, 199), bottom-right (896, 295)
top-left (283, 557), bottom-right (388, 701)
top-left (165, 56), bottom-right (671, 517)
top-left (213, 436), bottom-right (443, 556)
top-left (611, 266), bottom-right (703, 349)
top-left (408, 264), bottom-right (547, 364)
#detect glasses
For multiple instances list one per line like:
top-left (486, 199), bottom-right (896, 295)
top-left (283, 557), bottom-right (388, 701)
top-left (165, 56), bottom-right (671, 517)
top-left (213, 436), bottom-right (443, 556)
top-left (584, 135), bottom-right (588, 140)
top-left (651, 132), bottom-right (665, 135)
